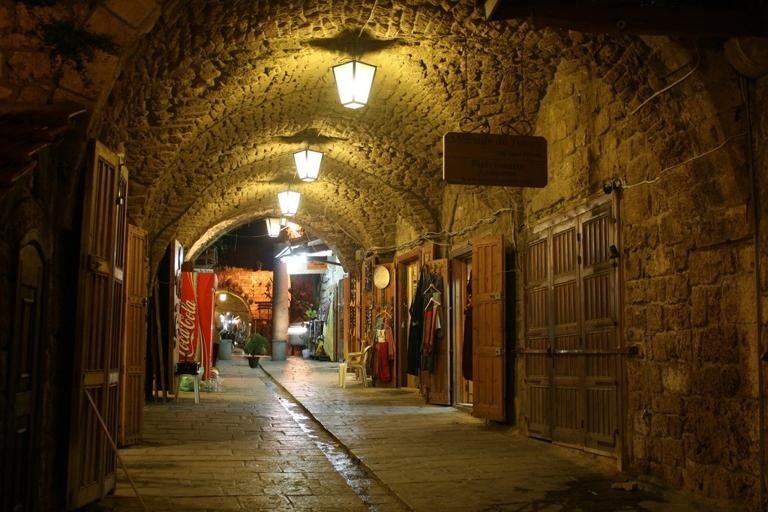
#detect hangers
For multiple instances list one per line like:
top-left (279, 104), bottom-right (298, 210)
top-left (423, 296), bottom-right (440, 312)
top-left (422, 279), bottom-right (441, 295)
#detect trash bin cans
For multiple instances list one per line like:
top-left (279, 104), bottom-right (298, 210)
top-left (219, 339), bottom-right (232, 360)
top-left (271, 340), bottom-right (287, 361)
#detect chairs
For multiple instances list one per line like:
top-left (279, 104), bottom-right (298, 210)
top-left (336, 345), bottom-right (371, 389)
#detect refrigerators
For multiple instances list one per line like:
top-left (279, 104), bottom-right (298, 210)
top-left (177, 271), bottom-right (218, 382)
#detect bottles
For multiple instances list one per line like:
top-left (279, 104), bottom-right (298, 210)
top-left (204, 378), bottom-right (224, 391)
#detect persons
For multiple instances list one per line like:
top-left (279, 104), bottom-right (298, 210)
top-left (212, 302), bottom-right (223, 369)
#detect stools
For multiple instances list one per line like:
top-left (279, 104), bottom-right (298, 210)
top-left (173, 372), bottom-right (200, 404)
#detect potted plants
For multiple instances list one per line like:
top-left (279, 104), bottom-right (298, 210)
top-left (244, 333), bottom-right (268, 369)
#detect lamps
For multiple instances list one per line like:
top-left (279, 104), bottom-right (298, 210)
top-left (292, 141), bottom-right (325, 183)
top-left (275, 191), bottom-right (299, 220)
top-left (264, 216), bottom-right (280, 238)
top-left (330, 39), bottom-right (377, 109)
